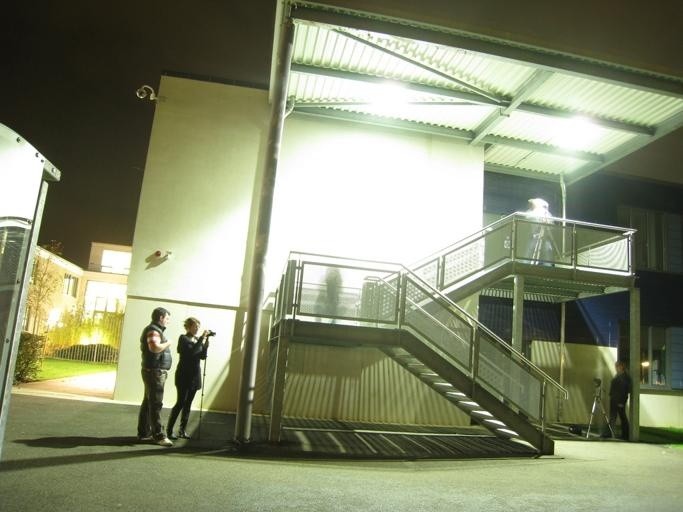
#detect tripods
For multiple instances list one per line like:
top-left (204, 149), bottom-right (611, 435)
top-left (585, 395), bottom-right (615, 439)
top-left (530, 225), bottom-right (565, 266)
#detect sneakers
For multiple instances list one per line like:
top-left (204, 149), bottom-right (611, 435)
top-left (137, 432), bottom-right (190, 447)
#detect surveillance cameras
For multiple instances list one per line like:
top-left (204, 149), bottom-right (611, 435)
top-left (154, 248), bottom-right (161, 257)
top-left (136, 88), bottom-right (147, 99)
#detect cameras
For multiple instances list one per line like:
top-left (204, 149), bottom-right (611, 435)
top-left (205, 328), bottom-right (216, 339)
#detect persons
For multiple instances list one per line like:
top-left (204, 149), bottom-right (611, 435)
top-left (166, 318), bottom-right (210, 440)
top-left (524, 198), bottom-right (555, 267)
top-left (137, 307), bottom-right (174, 446)
top-left (600, 360), bottom-right (632, 440)
